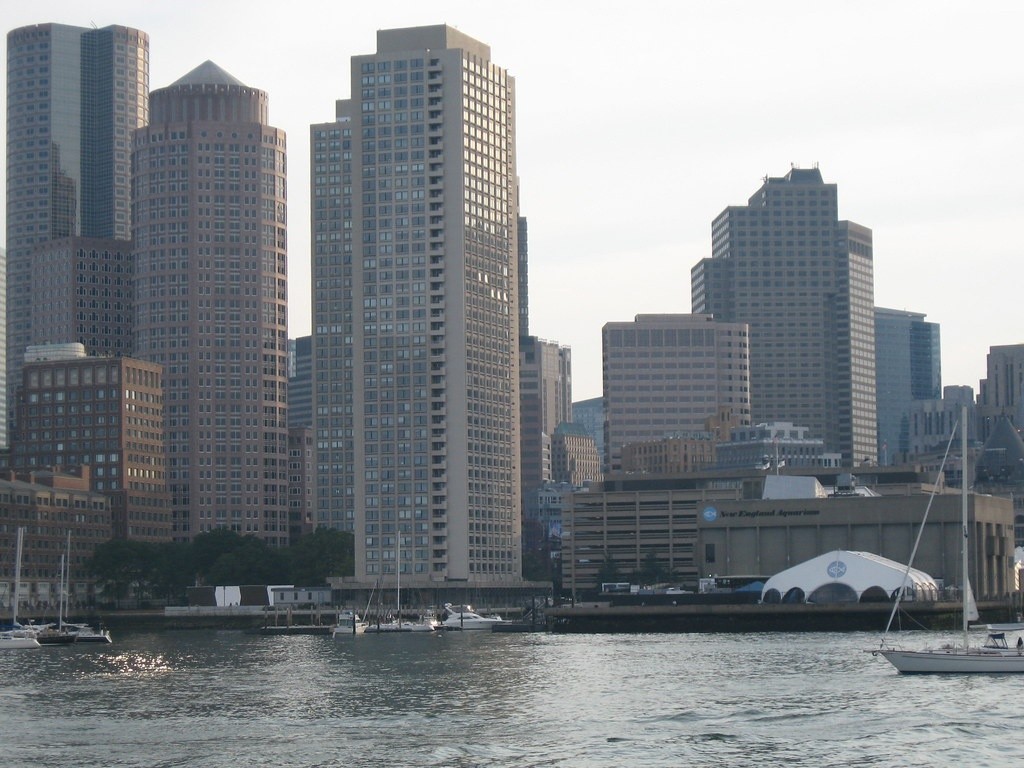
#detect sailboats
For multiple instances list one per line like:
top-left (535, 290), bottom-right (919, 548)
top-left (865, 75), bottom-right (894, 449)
top-left (0, 525), bottom-right (114, 650)
top-left (864, 404), bottom-right (1024, 675)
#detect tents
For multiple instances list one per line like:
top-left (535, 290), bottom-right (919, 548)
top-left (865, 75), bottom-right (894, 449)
top-left (761, 550), bottom-right (938, 604)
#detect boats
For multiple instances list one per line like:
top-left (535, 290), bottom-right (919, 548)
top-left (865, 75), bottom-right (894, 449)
top-left (243, 529), bottom-right (556, 634)
